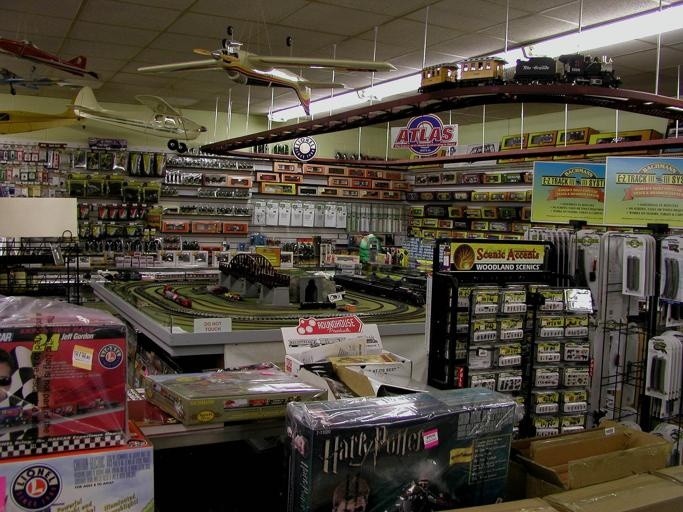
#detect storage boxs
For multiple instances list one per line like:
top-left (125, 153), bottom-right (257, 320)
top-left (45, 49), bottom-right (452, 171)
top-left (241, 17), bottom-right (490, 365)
top-left (1, 418), bottom-right (158, 512)
top-left (444, 423), bottom-right (681, 511)
top-left (1, 308), bottom-right (129, 440)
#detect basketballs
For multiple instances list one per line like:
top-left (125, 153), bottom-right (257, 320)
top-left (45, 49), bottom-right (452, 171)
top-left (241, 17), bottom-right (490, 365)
top-left (454, 245), bottom-right (475, 271)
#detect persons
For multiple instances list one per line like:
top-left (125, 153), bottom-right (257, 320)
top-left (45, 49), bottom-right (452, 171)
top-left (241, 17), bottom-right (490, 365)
top-left (0, 347), bottom-right (12, 406)
